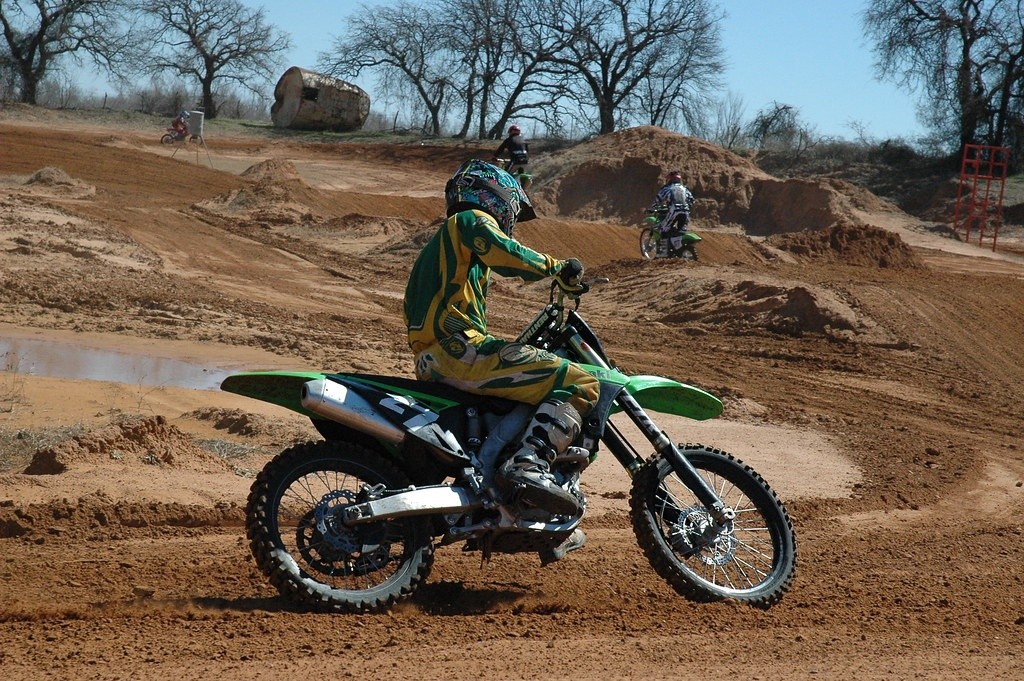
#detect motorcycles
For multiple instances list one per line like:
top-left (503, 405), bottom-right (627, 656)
top-left (639, 204), bottom-right (702, 260)
top-left (491, 156), bottom-right (532, 202)
top-left (160, 125), bottom-right (203, 147)
top-left (218, 260), bottom-right (798, 614)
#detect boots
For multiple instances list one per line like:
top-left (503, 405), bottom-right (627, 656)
top-left (495, 399), bottom-right (579, 514)
top-left (655, 238), bottom-right (668, 257)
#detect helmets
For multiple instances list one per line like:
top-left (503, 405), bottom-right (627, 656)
top-left (665, 171), bottom-right (682, 185)
top-left (444, 159), bottom-right (537, 241)
top-left (508, 125), bottom-right (521, 136)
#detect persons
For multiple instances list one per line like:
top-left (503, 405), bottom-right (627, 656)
top-left (493, 124), bottom-right (529, 175)
top-left (648, 171), bottom-right (695, 258)
top-left (171, 110), bottom-right (190, 137)
top-left (404, 159), bottom-right (600, 563)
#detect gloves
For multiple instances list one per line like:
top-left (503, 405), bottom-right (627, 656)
top-left (555, 257), bottom-right (584, 292)
top-left (649, 207), bottom-right (655, 214)
top-left (492, 152), bottom-right (498, 157)
